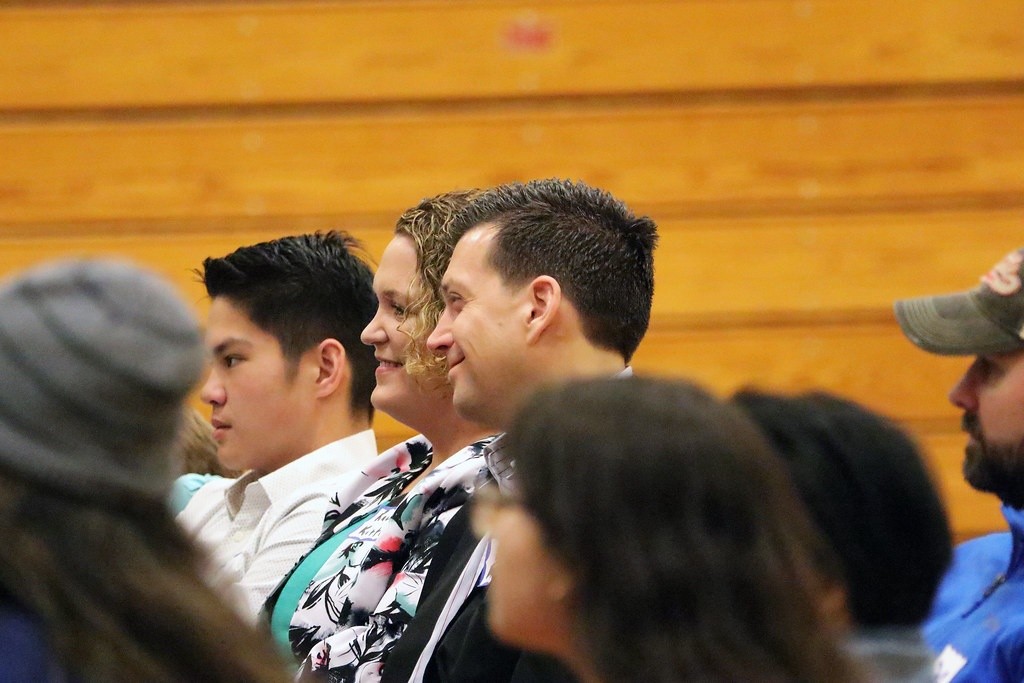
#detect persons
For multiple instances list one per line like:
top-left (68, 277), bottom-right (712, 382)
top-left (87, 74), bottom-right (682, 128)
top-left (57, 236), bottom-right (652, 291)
top-left (0, 178), bottom-right (1024, 682)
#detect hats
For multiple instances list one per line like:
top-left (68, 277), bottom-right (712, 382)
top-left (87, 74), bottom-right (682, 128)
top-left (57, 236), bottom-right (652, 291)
top-left (893, 249), bottom-right (1024, 356)
top-left (0, 262), bottom-right (204, 502)
top-left (730, 389), bottom-right (949, 627)
